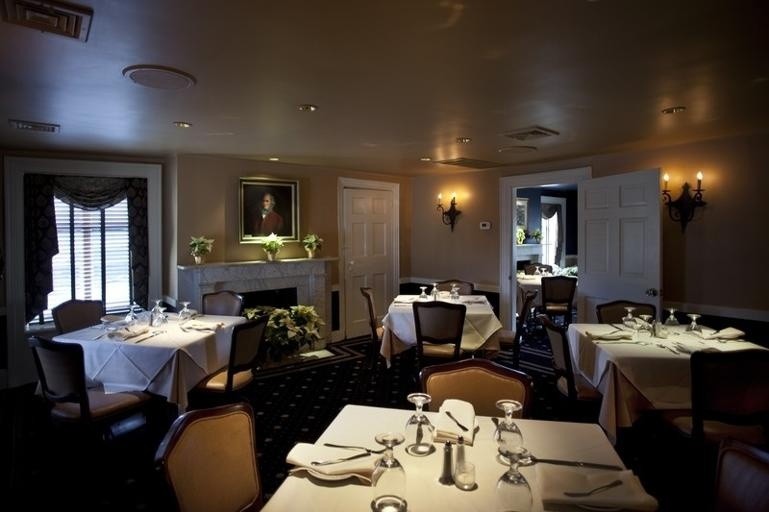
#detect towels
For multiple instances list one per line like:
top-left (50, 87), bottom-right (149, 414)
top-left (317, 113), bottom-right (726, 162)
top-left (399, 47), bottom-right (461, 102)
top-left (287, 441), bottom-right (381, 477)
top-left (107, 326), bottom-right (148, 341)
top-left (183, 320), bottom-right (224, 330)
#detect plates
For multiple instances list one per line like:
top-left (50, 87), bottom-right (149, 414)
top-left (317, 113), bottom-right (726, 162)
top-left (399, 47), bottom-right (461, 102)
top-left (308, 471), bottom-right (354, 483)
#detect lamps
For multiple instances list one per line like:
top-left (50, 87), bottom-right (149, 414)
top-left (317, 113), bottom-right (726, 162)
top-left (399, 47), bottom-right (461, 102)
top-left (661, 171), bottom-right (706, 234)
top-left (437, 192), bottom-right (462, 232)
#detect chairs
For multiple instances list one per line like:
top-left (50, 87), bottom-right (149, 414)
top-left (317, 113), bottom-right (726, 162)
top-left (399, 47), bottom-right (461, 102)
top-left (711, 437), bottom-right (768, 508)
top-left (486, 293), bottom-right (539, 367)
top-left (417, 358), bottom-right (535, 417)
top-left (52, 299), bottom-right (106, 334)
top-left (414, 302), bottom-right (466, 359)
top-left (393, 263), bottom-right (660, 323)
top-left (202, 289), bottom-right (244, 316)
top-left (543, 323), bottom-right (602, 418)
top-left (361, 286), bottom-right (412, 383)
top-left (671, 349), bottom-right (769, 481)
top-left (155, 400), bottom-right (268, 512)
top-left (29, 336), bottom-right (153, 447)
top-left (196, 315), bottom-right (270, 403)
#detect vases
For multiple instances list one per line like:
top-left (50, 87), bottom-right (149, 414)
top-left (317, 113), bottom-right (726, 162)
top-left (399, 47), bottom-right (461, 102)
top-left (270, 341), bottom-right (288, 359)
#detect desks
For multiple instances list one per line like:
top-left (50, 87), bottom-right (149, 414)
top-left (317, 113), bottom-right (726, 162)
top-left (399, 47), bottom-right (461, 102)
top-left (562, 323), bottom-right (767, 448)
top-left (373, 296), bottom-right (502, 370)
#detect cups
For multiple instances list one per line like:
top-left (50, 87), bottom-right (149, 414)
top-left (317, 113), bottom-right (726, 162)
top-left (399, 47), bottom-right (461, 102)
top-left (454, 460), bottom-right (477, 492)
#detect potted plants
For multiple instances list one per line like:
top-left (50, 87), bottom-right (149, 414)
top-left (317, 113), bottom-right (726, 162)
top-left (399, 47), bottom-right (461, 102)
top-left (302, 235), bottom-right (323, 258)
top-left (189, 236), bottom-right (215, 265)
top-left (262, 232), bottom-right (284, 261)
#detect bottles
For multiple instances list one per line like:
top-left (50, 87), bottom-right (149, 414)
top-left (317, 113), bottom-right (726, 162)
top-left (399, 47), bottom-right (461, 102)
top-left (451, 434), bottom-right (471, 485)
top-left (438, 439), bottom-right (453, 484)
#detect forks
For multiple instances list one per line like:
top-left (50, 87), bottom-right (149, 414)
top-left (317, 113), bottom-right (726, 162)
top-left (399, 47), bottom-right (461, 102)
top-left (324, 442), bottom-right (389, 456)
top-left (492, 417), bottom-right (507, 446)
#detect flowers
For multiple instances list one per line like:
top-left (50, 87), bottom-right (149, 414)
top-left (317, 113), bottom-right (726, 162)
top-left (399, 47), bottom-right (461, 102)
top-left (244, 305), bottom-right (325, 352)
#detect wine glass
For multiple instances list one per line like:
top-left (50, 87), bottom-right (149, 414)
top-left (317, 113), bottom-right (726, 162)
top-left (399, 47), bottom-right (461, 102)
top-left (492, 397), bottom-right (527, 459)
top-left (587, 301), bottom-right (742, 355)
top-left (493, 453), bottom-right (536, 511)
top-left (367, 431), bottom-right (409, 512)
top-left (90, 293), bottom-right (220, 344)
top-left (405, 393), bottom-right (437, 455)
top-left (517, 259), bottom-right (583, 282)
top-left (393, 270), bottom-right (486, 311)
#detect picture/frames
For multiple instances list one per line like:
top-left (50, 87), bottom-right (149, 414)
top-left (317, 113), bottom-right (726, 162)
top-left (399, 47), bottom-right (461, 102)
top-left (516, 198), bottom-right (529, 231)
top-left (237, 177), bottom-right (300, 244)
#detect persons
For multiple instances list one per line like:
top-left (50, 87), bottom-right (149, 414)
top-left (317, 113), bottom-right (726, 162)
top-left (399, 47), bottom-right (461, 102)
top-left (251, 193), bottom-right (284, 236)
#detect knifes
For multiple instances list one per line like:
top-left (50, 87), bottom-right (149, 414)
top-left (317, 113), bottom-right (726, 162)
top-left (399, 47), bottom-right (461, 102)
top-left (310, 451), bottom-right (376, 470)
top-left (531, 456), bottom-right (624, 472)
top-left (445, 409), bottom-right (469, 433)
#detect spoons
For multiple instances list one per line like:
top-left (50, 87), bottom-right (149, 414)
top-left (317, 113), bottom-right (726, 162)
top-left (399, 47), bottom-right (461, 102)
top-left (558, 480), bottom-right (623, 497)
